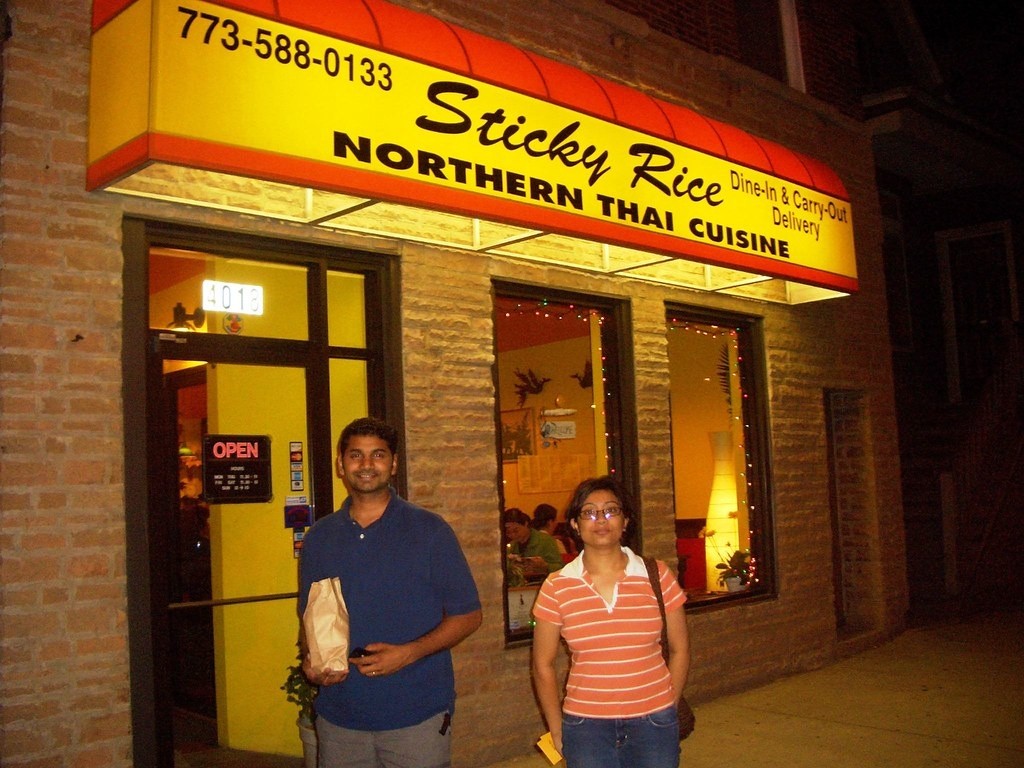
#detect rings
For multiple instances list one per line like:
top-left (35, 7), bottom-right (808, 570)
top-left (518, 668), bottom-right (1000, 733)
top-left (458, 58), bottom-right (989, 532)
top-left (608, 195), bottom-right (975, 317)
top-left (372, 672), bottom-right (376, 676)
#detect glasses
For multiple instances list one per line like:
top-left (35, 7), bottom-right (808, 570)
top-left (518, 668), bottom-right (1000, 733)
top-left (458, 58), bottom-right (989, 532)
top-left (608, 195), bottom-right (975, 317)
top-left (579, 507), bottom-right (624, 519)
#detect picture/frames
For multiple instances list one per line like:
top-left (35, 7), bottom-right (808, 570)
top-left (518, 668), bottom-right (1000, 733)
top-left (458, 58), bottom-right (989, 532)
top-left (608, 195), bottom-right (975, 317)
top-left (500, 407), bottom-right (538, 465)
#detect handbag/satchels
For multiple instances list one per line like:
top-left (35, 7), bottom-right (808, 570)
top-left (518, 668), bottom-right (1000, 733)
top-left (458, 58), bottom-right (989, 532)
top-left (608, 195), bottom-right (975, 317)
top-left (642, 555), bottom-right (696, 743)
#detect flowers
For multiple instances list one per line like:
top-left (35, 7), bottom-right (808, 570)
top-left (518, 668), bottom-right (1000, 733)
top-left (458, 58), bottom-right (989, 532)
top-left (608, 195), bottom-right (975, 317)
top-left (506, 544), bottom-right (528, 587)
top-left (698, 512), bottom-right (739, 562)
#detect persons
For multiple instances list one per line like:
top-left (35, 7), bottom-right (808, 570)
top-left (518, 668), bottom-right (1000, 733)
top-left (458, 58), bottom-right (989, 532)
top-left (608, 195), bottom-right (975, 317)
top-left (531, 475), bottom-right (691, 768)
top-left (503, 504), bottom-right (584, 581)
top-left (296, 416), bottom-right (483, 768)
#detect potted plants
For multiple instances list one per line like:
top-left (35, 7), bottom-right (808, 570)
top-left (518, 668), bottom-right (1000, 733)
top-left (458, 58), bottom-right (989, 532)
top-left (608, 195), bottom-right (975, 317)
top-left (280, 639), bottom-right (319, 768)
top-left (715, 550), bottom-right (750, 592)
